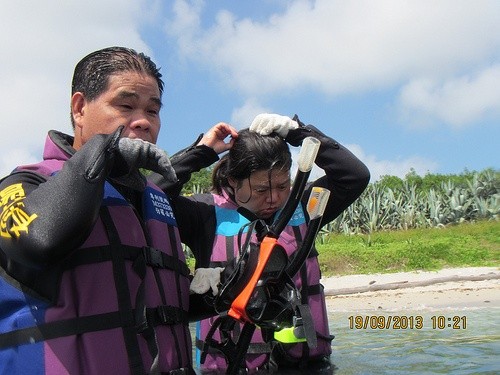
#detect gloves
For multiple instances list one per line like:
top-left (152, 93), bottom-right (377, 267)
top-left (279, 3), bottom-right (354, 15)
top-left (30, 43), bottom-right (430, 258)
top-left (190, 267), bottom-right (225, 296)
top-left (117, 135), bottom-right (177, 193)
top-left (248, 112), bottom-right (298, 141)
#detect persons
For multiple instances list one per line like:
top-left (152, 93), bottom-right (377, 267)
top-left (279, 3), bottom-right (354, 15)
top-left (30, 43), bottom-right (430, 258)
top-left (0, 46), bottom-right (193, 375)
top-left (161, 114), bottom-right (371, 374)
